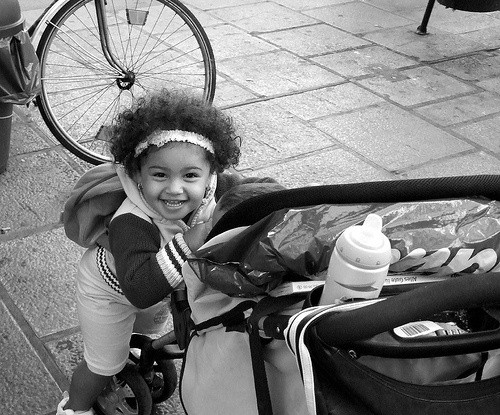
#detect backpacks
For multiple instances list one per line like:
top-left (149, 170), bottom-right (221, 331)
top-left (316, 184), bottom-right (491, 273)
top-left (60, 161), bottom-right (126, 253)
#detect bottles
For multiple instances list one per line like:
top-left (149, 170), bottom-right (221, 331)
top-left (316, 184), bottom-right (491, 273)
top-left (318, 213), bottom-right (393, 307)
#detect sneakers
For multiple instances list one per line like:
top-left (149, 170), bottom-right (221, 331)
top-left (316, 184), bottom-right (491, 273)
top-left (55, 390), bottom-right (96, 415)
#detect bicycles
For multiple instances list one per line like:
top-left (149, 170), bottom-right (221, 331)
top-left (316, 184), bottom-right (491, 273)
top-left (22, 0), bottom-right (217, 165)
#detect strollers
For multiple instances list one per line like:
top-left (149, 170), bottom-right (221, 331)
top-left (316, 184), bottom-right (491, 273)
top-left (74, 165), bottom-right (500, 414)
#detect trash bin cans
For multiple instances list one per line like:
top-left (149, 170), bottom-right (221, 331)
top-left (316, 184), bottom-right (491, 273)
top-left (0, 0), bottom-right (41, 174)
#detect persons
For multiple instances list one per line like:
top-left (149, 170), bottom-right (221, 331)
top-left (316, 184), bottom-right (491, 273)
top-left (56, 88), bottom-right (286, 415)
